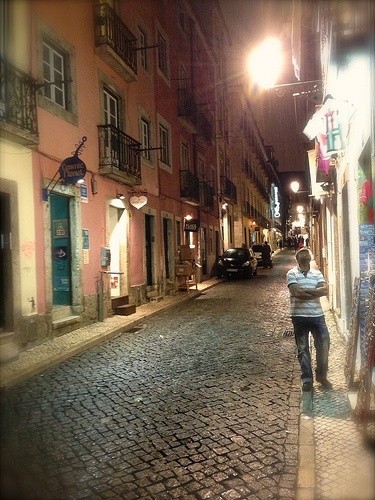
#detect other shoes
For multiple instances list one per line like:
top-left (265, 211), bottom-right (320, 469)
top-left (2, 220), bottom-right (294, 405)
top-left (302, 379), bottom-right (313, 391)
top-left (269, 266), bottom-right (273, 269)
top-left (315, 375), bottom-right (333, 389)
top-left (263, 266), bottom-right (268, 269)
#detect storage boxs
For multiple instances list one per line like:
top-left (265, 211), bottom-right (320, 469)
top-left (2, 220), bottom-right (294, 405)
top-left (182, 261), bottom-right (202, 272)
top-left (180, 245), bottom-right (195, 260)
top-left (175, 265), bottom-right (191, 275)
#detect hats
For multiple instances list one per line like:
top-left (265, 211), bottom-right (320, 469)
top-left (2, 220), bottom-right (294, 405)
top-left (295, 248), bottom-right (310, 257)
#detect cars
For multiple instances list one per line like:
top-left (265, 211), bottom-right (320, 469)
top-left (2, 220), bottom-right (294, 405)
top-left (250, 242), bottom-right (275, 264)
top-left (216, 246), bottom-right (258, 279)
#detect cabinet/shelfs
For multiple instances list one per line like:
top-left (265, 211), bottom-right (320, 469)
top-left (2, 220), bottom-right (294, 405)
top-left (174, 272), bottom-right (197, 292)
